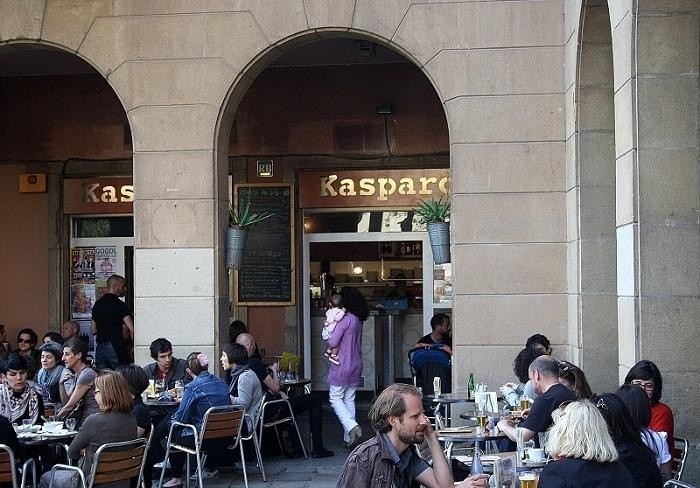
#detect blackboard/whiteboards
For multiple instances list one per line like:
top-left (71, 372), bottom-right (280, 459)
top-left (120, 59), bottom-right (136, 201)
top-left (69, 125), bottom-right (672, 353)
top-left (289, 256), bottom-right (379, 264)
top-left (234, 183), bottom-right (294, 305)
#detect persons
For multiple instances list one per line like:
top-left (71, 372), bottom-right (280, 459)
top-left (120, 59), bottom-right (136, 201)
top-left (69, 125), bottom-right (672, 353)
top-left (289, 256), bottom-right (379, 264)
top-left (233, 330), bottom-right (334, 459)
top-left (337, 381), bottom-right (492, 487)
top-left (386, 274), bottom-right (419, 308)
top-left (321, 294), bottom-right (348, 367)
top-left (0, 318), bottom-right (265, 488)
top-left (229, 319), bottom-right (262, 357)
top-left (323, 272), bottom-right (337, 302)
top-left (327, 286), bottom-right (370, 448)
top-left (494, 334), bottom-right (676, 486)
top-left (415, 312), bottom-right (453, 357)
top-left (90, 272), bottom-right (135, 371)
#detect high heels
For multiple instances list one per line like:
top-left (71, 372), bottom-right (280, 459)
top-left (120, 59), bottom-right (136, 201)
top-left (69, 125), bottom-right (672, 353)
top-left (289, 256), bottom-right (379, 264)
top-left (346, 426), bottom-right (363, 448)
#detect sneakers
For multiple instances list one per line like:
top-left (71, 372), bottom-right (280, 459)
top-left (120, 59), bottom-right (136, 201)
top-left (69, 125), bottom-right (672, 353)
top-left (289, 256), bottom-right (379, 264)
top-left (329, 353), bottom-right (340, 365)
top-left (324, 350), bottom-right (331, 357)
top-left (313, 450), bottom-right (335, 458)
top-left (190, 469), bottom-right (220, 480)
top-left (153, 459), bottom-right (171, 469)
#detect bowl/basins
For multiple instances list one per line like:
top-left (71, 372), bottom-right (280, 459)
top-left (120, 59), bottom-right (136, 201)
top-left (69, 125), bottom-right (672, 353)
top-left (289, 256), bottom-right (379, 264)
top-left (44, 421), bottom-right (63, 434)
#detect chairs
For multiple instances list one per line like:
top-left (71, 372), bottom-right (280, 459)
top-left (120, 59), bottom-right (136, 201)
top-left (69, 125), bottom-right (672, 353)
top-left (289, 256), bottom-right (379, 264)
top-left (665, 435), bottom-right (689, 479)
top-left (662, 475), bottom-right (695, 487)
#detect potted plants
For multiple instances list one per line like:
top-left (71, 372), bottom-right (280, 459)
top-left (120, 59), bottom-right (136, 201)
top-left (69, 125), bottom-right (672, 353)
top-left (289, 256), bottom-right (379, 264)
top-left (412, 190), bottom-right (452, 267)
top-left (226, 193), bottom-right (277, 270)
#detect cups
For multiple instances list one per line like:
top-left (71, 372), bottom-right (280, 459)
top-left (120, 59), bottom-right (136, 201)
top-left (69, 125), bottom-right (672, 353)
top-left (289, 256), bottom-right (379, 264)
top-left (476, 413), bottom-right (486, 432)
top-left (520, 395), bottom-right (528, 411)
top-left (155, 380), bottom-right (163, 394)
top-left (175, 380), bottom-right (184, 400)
top-left (519, 471), bottom-right (538, 488)
top-left (529, 449), bottom-right (542, 461)
top-left (470, 390), bottom-right (476, 399)
top-left (433, 377), bottom-right (442, 394)
top-left (65, 417), bottom-right (76, 432)
top-left (23, 419), bottom-right (34, 434)
top-left (499, 409), bottom-right (509, 421)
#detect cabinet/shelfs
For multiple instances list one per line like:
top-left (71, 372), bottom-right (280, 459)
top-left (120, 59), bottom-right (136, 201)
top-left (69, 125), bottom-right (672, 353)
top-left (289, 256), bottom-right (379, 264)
top-left (326, 273), bottom-right (422, 306)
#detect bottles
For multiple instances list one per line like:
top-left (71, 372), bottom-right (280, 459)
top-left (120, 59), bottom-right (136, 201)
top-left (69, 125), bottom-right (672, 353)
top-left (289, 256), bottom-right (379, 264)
top-left (286, 361), bottom-right (300, 380)
top-left (513, 448), bottom-right (522, 460)
top-left (467, 373), bottom-right (474, 395)
top-left (469, 451), bottom-right (485, 478)
top-left (14, 423), bottom-right (18, 433)
top-left (484, 392), bottom-right (494, 431)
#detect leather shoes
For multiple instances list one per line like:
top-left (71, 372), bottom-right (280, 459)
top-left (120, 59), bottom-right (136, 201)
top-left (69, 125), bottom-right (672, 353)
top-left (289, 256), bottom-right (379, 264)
top-left (157, 482), bottom-right (183, 488)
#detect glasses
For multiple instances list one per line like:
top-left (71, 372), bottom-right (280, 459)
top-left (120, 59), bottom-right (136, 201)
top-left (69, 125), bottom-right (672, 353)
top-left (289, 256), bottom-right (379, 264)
top-left (589, 393), bottom-right (608, 413)
top-left (559, 400), bottom-right (572, 415)
top-left (18, 338), bottom-right (32, 343)
top-left (632, 381), bottom-right (656, 392)
